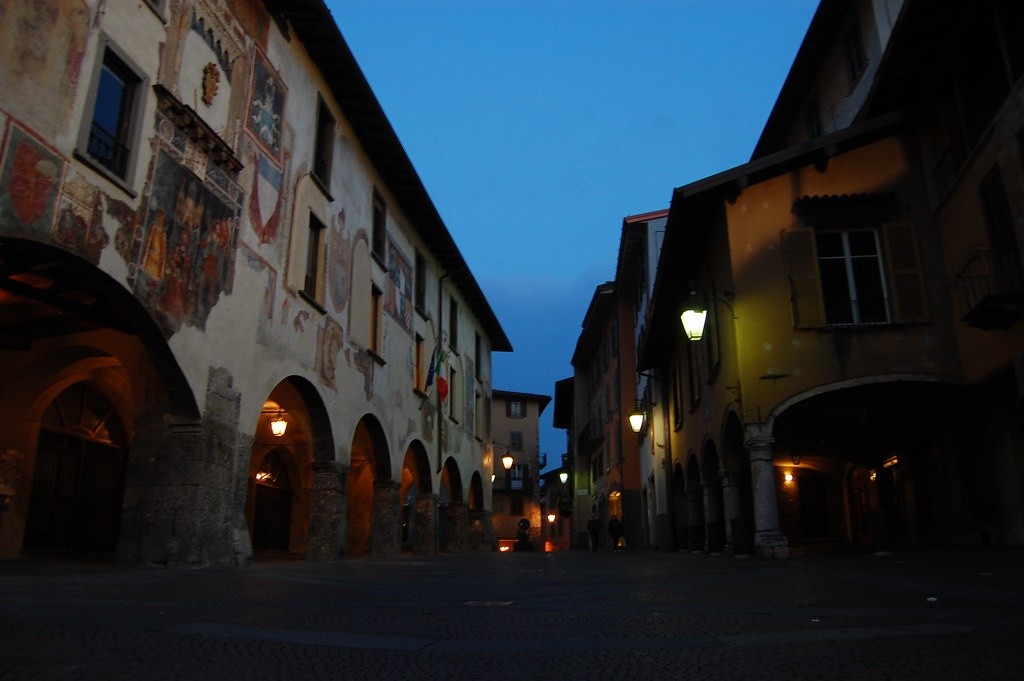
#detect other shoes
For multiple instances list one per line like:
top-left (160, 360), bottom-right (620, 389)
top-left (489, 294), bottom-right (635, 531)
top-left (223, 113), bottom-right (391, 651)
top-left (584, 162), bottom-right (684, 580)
top-left (614, 550), bottom-right (617, 553)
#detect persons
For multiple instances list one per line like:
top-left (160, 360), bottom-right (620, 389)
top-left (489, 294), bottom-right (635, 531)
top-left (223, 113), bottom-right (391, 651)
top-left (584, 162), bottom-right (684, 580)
top-left (608, 515), bottom-right (623, 552)
top-left (587, 515), bottom-right (601, 553)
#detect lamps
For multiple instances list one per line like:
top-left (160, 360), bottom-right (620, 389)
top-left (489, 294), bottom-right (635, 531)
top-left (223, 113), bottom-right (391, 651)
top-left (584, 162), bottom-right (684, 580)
top-left (680, 283), bottom-right (735, 341)
top-left (269, 413), bottom-right (288, 437)
top-left (493, 441), bottom-right (514, 469)
top-left (557, 467), bottom-right (572, 483)
top-left (628, 398), bottom-right (657, 433)
top-left (546, 514), bottom-right (556, 522)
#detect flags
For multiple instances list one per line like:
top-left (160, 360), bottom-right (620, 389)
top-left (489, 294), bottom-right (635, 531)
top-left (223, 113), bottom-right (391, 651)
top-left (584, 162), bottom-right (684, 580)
top-left (424, 350), bottom-right (448, 402)
